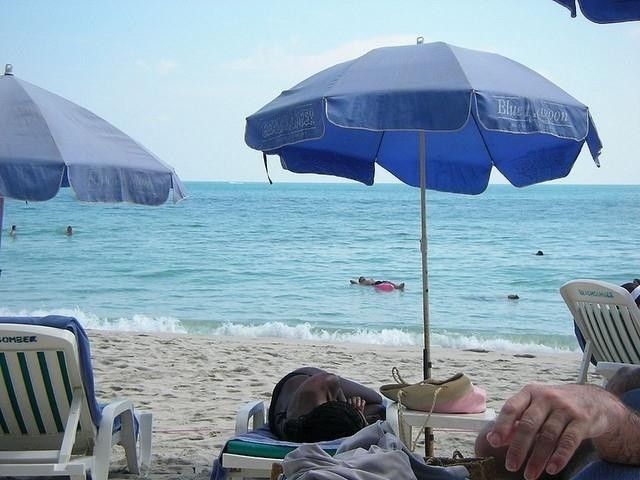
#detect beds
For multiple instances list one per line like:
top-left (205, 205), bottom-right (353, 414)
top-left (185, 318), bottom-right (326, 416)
top-left (222, 400), bottom-right (399, 479)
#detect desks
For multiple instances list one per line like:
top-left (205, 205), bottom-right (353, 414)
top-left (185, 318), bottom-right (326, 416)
top-left (400, 408), bottom-right (496, 453)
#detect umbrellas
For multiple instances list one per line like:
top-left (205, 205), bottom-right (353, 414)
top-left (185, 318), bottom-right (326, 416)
top-left (245, 36), bottom-right (602, 460)
top-left (552, 0), bottom-right (640, 24)
top-left (0, 64), bottom-right (191, 266)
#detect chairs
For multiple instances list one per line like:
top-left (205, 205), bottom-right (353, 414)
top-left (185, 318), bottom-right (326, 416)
top-left (1, 315), bottom-right (153, 480)
top-left (559, 279), bottom-right (640, 388)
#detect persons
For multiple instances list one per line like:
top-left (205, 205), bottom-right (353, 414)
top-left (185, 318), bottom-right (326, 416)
top-left (66, 225), bottom-right (72, 235)
top-left (615, 282), bottom-right (640, 311)
top-left (9, 225), bottom-right (16, 236)
top-left (358, 277), bottom-right (405, 289)
top-left (267, 367), bottom-right (382, 444)
top-left (508, 294), bottom-right (519, 299)
top-left (472, 367), bottom-right (640, 479)
top-left (535, 251), bottom-right (543, 255)
top-left (633, 279), bottom-right (639, 283)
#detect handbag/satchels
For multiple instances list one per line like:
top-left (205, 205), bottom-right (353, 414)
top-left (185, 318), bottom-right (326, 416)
top-left (379, 367), bottom-right (487, 453)
